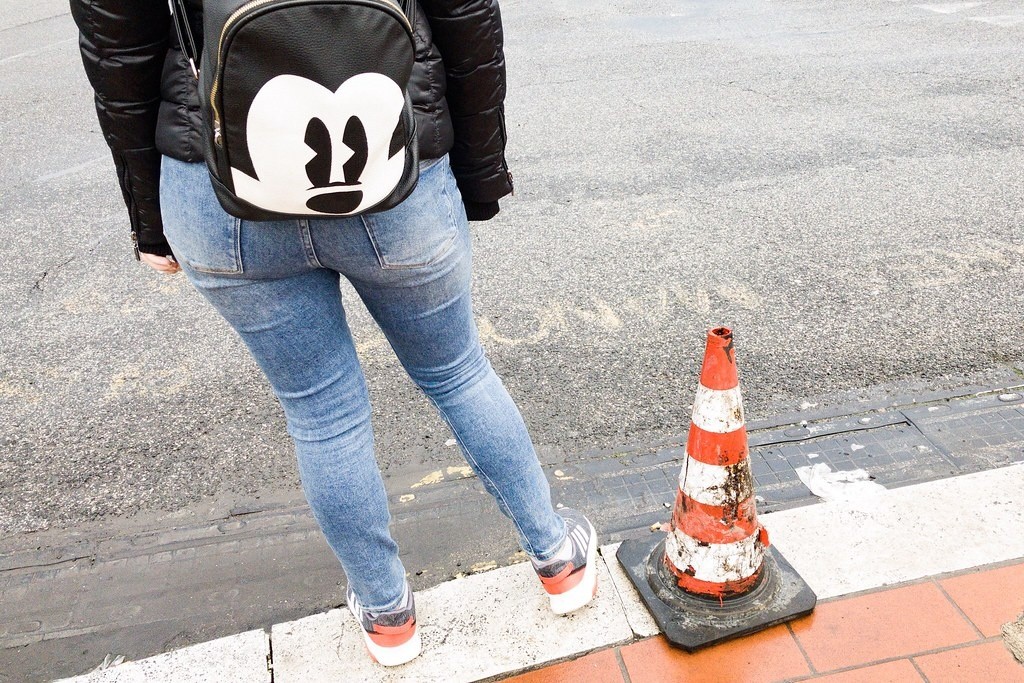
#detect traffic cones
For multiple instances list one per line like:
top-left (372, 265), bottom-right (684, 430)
top-left (616, 326), bottom-right (817, 653)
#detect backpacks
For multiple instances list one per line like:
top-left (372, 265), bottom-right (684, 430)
top-left (168, 0), bottom-right (420, 221)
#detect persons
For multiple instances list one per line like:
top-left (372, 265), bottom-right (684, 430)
top-left (70, 0), bottom-right (598, 667)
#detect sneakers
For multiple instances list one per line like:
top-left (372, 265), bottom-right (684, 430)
top-left (531, 508), bottom-right (597, 615)
top-left (347, 578), bottom-right (422, 666)
